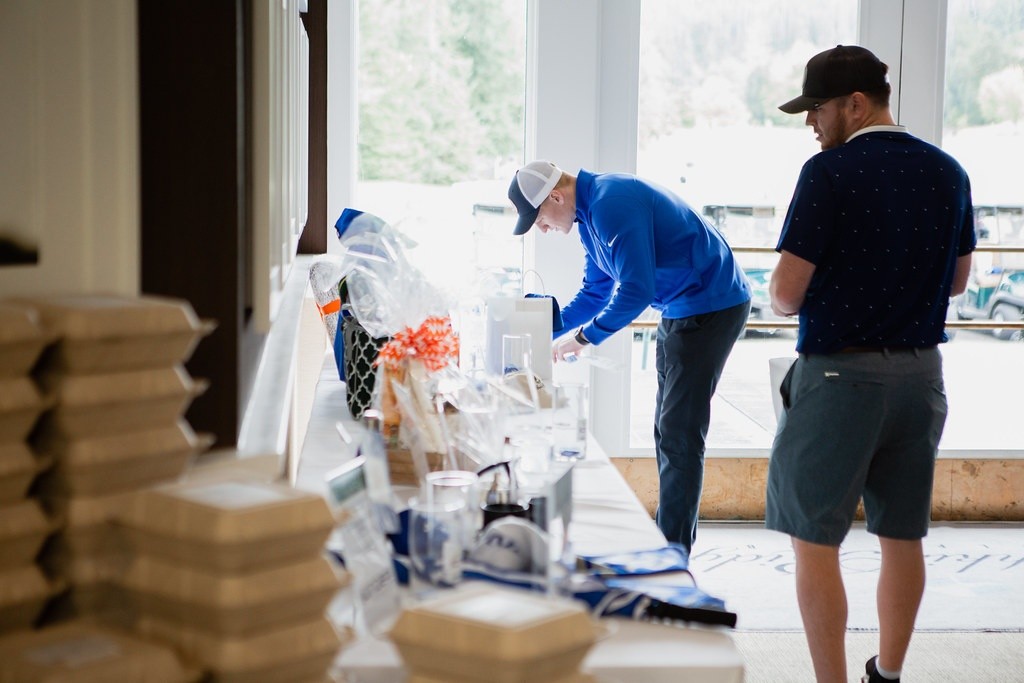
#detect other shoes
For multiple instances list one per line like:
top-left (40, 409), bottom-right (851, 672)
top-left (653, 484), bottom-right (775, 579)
top-left (861, 656), bottom-right (900, 683)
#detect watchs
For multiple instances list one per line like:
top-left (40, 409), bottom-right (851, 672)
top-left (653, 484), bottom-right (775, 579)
top-left (575, 325), bottom-right (589, 345)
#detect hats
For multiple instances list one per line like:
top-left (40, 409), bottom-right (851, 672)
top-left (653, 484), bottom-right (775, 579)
top-left (507, 161), bottom-right (563, 235)
top-left (777, 45), bottom-right (890, 114)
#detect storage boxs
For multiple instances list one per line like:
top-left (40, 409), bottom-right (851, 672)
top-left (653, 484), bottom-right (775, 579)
top-left (0, 294), bottom-right (592, 683)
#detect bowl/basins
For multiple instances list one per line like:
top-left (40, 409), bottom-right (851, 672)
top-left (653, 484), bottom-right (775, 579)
top-left (382, 578), bottom-right (608, 683)
top-left (0, 289), bottom-right (355, 683)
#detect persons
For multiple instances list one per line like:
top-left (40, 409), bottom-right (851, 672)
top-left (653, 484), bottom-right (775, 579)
top-left (508, 161), bottom-right (753, 558)
top-left (763, 47), bottom-right (978, 683)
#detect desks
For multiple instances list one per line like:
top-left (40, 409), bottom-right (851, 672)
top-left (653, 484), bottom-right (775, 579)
top-left (295, 380), bottom-right (750, 683)
